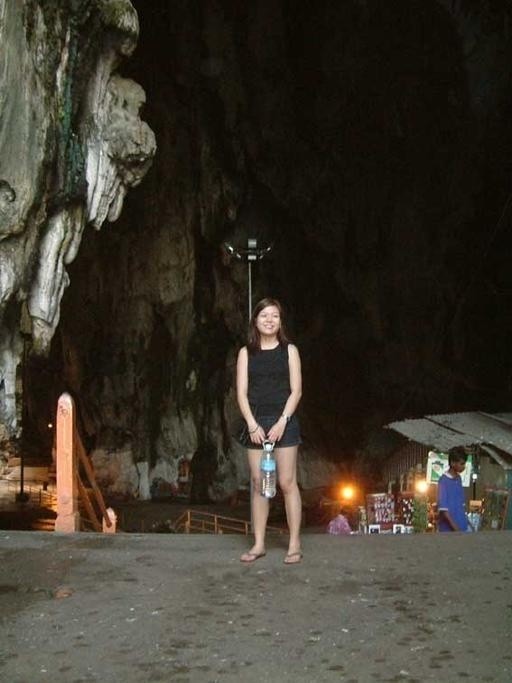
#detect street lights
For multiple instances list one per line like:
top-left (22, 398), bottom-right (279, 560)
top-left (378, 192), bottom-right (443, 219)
top-left (223, 237), bottom-right (271, 344)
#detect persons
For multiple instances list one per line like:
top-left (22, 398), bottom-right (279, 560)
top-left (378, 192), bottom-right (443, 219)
top-left (437, 446), bottom-right (475, 533)
top-left (326, 505), bottom-right (355, 535)
top-left (235, 297), bottom-right (302, 564)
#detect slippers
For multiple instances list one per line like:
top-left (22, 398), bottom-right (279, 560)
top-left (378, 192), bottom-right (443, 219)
top-left (284, 549), bottom-right (303, 564)
top-left (241, 549), bottom-right (266, 562)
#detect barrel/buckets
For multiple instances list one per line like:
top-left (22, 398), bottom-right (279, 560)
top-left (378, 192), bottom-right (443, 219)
top-left (258, 439), bottom-right (277, 499)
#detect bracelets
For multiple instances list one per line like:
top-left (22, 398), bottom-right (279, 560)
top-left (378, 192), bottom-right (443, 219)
top-left (246, 422), bottom-right (259, 433)
top-left (282, 414), bottom-right (291, 422)
